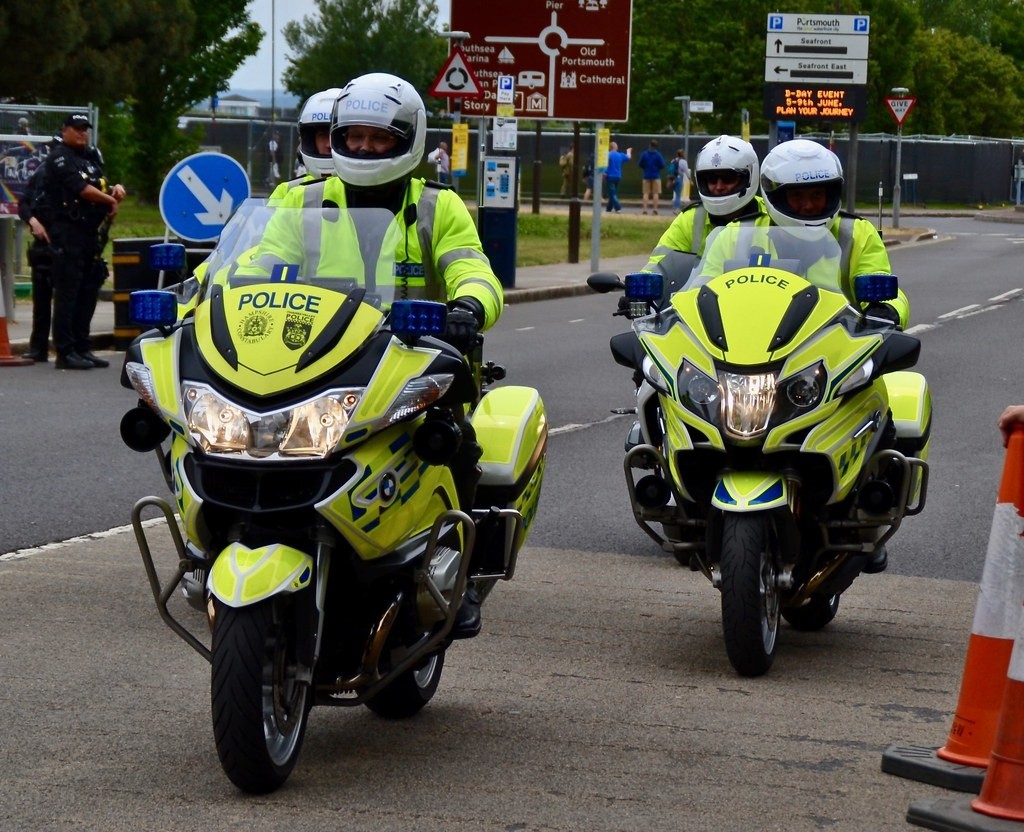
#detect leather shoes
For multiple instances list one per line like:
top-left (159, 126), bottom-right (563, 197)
top-left (454, 580), bottom-right (482, 639)
top-left (55, 351), bottom-right (110, 370)
top-left (862, 544), bottom-right (888, 574)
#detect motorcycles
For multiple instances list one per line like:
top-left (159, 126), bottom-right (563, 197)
top-left (591, 224), bottom-right (931, 680)
top-left (122, 204), bottom-right (551, 793)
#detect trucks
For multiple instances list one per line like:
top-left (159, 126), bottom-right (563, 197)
top-left (0, 103), bottom-right (99, 213)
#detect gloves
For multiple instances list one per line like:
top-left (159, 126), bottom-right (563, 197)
top-left (866, 303), bottom-right (899, 329)
top-left (447, 306), bottom-right (477, 340)
top-left (618, 295), bottom-right (652, 319)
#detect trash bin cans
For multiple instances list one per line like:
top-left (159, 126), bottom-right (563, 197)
top-left (112, 238), bottom-right (178, 351)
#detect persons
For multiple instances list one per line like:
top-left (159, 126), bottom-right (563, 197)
top-left (617, 134), bottom-right (910, 574)
top-left (560, 143), bottom-right (574, 199)
top-left (602, 141), bottom-right (632, 214)
top-left (17, 114), bottom-right (126, 371)
top-left (996, 405), bottom-right (1024, 448)
top-left (582, 149), bottom-right (595, 200)
top-left (193, 72), bottom-right (503, 639)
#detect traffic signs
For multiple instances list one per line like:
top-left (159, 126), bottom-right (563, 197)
top-left (764, 33), bottom-right (870, 85)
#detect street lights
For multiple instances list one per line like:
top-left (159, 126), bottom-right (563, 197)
top-left (673, 95), bottom-right (692, 169)
top-left (440, 29), bottom-right (470, 118)
top-left (890, 87), bottom-right (913, 228)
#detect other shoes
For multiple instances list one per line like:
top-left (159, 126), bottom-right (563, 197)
top-left (22, 349), bottom-right (48, 362)
top-left (642, 210), bottom-right (647, 214)
top-left (653, 210), bottom-right (659, 215)
top-left (673, 208), bottom-right (682, 215)
top-left (604, 209), bottom-right (621, 214)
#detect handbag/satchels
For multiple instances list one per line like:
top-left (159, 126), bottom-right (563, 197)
top-left (428, 148), bottom-right (442, 164)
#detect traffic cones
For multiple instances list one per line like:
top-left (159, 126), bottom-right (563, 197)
top-left (881, 424), bottom-right (1024, 797)
top-left (904, 611), bottom-right (1024, 832)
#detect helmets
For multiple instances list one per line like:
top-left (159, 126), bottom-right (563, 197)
top-left (760, 139), bottom-right (844, 242)
top-left (330, 73), bottom-right (427, 187)
top-left (300, 88), bottom-right (343, 179)
top-left (694, 134), bottom-right (759, 215)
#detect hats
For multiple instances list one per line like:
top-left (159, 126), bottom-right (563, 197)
top-left (64, 113), bottom-right (94, 130)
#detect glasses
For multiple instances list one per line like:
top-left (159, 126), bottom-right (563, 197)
top-left (707, 173), bottom-right (736, 184)
top-left (341, 131), bottom-right (395, 145)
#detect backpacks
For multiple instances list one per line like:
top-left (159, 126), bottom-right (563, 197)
top-left (667, 158), bottom-right (682, 176)
